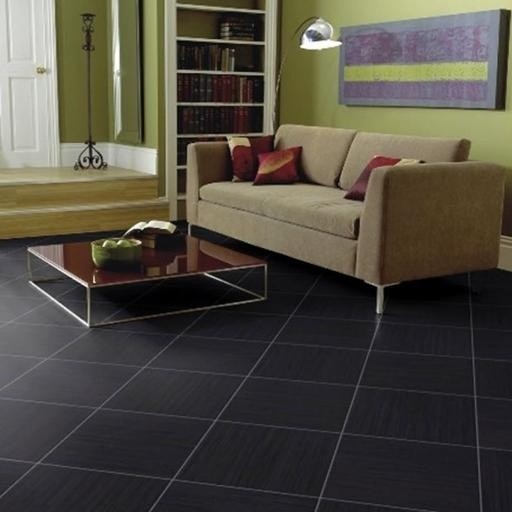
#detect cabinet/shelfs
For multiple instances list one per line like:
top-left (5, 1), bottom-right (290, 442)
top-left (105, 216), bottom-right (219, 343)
top-left (166, 1), bottom-right (277, 221)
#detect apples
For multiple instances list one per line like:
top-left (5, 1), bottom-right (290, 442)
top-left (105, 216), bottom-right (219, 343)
top-left (102, 240), bottom-right (118, 248)
top-left (117, 240), bottom-right (132, 247)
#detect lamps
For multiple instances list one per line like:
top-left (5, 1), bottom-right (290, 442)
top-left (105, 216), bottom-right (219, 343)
top-left (279, 16), bottom-right (343, 91)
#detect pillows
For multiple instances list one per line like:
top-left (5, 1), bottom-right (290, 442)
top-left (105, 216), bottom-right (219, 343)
top-left (344, 156), bottom-right (424, 200)
top-left (225, 135), bottom-right (303, 185)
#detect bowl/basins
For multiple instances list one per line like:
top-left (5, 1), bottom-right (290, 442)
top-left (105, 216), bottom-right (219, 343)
top-left (91, 237), bottom-right (143, 271)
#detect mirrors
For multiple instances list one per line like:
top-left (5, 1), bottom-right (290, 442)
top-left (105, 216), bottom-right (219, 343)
top-left (106, 0), bottom-right (143, 145)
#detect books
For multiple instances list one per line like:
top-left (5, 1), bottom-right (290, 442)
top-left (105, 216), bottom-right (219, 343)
top-left (177, 15), bottom-right (264, 166)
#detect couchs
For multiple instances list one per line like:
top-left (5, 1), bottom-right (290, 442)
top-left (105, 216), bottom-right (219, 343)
top-left (187, 123), bottom-right (506, 315)
